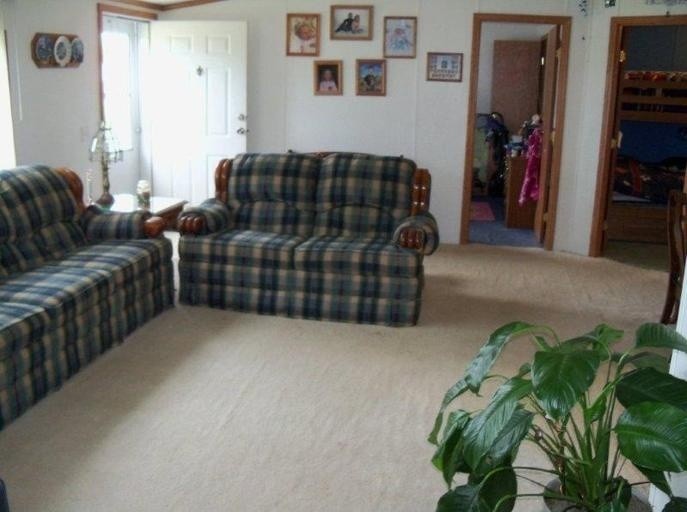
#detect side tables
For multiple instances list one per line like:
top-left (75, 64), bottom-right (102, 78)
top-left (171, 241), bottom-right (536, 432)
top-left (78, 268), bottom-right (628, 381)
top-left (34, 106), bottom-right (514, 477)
top-left (110, 193), bottom-right (189, 239)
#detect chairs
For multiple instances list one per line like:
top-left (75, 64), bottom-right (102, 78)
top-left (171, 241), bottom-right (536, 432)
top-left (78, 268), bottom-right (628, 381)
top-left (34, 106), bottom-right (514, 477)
top-left (659, 190), bottom-right (686, 324)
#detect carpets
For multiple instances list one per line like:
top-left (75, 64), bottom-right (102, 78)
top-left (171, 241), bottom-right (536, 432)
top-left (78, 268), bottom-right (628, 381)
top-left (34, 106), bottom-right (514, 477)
top-left (468, 202), bottom-right (496, 222)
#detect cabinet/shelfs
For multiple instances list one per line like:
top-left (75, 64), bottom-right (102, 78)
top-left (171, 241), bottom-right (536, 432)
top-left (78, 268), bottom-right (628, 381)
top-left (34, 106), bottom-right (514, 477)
top-left (504, 151), bottom-right (535, 229)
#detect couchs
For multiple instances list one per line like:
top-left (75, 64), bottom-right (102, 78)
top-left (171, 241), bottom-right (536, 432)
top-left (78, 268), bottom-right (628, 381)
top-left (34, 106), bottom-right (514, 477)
top-left (176, 150), bottom-right (440, 326)
top-left (0, 163), bottom-right (176, 430)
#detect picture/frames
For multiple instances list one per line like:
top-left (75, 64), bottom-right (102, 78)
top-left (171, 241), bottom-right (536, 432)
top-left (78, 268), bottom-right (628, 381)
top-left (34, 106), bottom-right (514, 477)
top-left (383, 16), bottom-right (417, 59)
top-left (329, 5), bottom-right (374, 41)
top-left (286, 13), bottom-right (321, 56)
top-left (355, 59), bottom-right (387, 96)
top-left (425, 52), bottom-right (463, 82)
top-left (312, 60), bottom-right (343, 95)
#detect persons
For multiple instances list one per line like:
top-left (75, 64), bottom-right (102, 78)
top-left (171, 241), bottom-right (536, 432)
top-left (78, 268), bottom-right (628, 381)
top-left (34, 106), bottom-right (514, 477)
top-left (335, 11), bottom-right (353, 32)
top-left (351, 14), bottom-right (364, 34)
top-left (319, 68), bottom-right (338, 90)
top-left (291, 21), bottom-right (318, 54)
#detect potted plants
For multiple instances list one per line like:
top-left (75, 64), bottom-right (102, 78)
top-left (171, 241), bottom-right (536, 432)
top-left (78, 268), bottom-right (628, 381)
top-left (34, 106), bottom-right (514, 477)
top-left (428, 321), bottom-right (687, 512)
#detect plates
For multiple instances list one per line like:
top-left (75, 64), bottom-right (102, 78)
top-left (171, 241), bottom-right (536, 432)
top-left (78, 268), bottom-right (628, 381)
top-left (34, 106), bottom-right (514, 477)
top-left (34, 35), bottom-right (84, 67)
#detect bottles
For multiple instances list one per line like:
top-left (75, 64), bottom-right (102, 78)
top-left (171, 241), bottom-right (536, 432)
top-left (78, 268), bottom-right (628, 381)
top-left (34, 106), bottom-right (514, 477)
top-left (134, 179), bottom-right (151, 210)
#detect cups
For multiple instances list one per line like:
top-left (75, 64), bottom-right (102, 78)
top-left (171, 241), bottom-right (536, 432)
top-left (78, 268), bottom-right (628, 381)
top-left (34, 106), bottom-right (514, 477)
top-left (510, 134), bottom-right (523, 145)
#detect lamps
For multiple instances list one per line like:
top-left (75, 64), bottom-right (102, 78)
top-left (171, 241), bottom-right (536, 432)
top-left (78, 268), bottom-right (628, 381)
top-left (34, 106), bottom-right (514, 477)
top-left (87, 123), bottom-right (123, 204)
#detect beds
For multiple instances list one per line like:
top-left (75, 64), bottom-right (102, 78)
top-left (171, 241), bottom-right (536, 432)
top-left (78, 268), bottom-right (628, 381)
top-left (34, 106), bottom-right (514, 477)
top-left (604, 70), bottom-right (687, 245)
